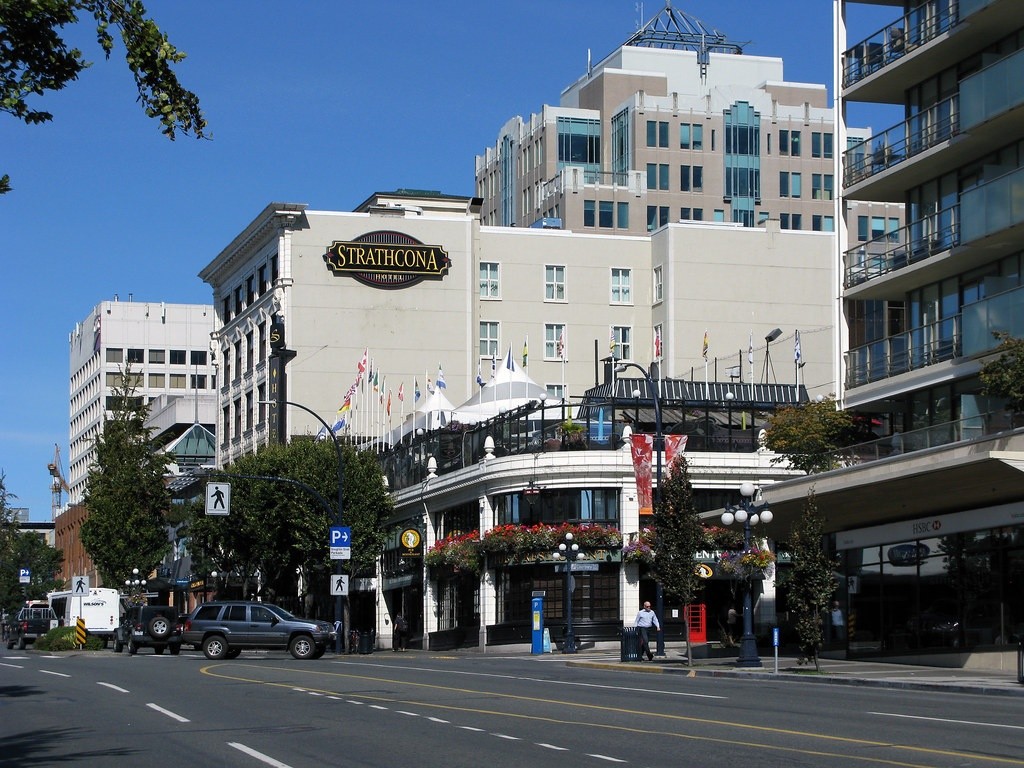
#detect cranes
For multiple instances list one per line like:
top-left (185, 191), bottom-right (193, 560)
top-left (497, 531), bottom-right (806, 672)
top-left (47, 442), bottom-right (69, 522)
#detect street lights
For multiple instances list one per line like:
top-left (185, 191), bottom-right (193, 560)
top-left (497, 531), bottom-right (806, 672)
top-left (257, 398), bottom-right (345, 655)
top-left (613, 362), bottom-right (665, 656)
top-left (553, 531), bottom-right (585, 655)
top-left (124, 567), bottom-right (148, 605)
top-left (720, 482), bottom-right (775, 668)
top-left (195, 564), bottom-right (218, 603)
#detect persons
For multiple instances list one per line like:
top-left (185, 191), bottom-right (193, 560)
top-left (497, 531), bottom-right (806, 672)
top-left (635, 602), bottom-right (661, 661)
top-left (725, 604), bottom-right (743, 642)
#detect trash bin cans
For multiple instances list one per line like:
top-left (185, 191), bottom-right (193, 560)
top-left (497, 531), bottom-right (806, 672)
top-left (620, 627), bottom-right (643, 662)
top-left (359, 633), bottom-right (373, 655)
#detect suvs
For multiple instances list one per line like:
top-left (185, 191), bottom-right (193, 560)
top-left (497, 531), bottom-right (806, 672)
top-left (181, 600), bottom-right (339, 660)
top-left (113, 606), bottom-right (182, 655)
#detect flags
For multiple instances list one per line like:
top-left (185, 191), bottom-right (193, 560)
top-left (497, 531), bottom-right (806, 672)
top-left (610, 327), bottom-right (617, 357)
top-left (794, 331), bottom-right (803, 367)
top-left (656, 328), bottom-right (660, 358)
top-left (558, 331), bottom-right (567, 362)
top-left (703, 333), bottom-right (709, 361)
top-left (331, 348), bottom-right (446, 433)
top-left (749, 330), bottom-right (753, 364)
top-left (477, 337), bottom-right (529, 388)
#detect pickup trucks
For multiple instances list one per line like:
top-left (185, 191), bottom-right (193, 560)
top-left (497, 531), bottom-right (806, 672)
top-left (6, 607), bottom-right (58, 651)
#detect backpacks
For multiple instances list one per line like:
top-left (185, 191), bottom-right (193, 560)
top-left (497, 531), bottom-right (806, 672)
top-left (398, 617), bottom-right (407, 631)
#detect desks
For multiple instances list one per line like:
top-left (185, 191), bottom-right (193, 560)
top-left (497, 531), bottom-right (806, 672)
top-left (871, 153), bottom-right (902, 174)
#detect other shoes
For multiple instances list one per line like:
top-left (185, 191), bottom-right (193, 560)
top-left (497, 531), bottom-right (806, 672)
top-left (649, 655), bottom-right (653, 661)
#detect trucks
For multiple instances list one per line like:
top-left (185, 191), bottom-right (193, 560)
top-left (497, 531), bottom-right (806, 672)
top-left (48, 587), bottom-right (121, 648)
top-left (23, 600), bottom-right (48, 609)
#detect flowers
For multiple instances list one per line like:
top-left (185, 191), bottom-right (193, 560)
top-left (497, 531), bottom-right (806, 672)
top-left (423, 522), bottom-right (777, 583)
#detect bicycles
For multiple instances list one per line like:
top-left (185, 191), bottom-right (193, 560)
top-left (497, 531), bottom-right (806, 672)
top-left (349, 628), bottom-right (360, 654)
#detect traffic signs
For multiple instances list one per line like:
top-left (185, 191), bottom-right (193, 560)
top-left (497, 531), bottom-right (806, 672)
top-left (18, 565), bottom-right (31, 584)
top-left (328, 527), bottom-right (351, 561)
